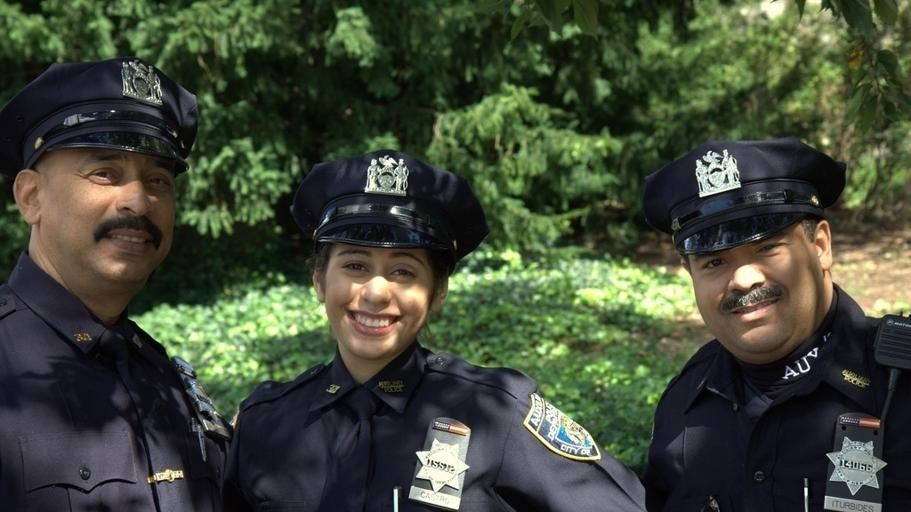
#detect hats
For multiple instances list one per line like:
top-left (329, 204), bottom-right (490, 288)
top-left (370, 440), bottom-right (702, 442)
top-left (643, 139), bottom-right (846, 255)
top-left (290, 150), bottom-right (488, 275)
top-left (0, 57), bottom-right (198, 179)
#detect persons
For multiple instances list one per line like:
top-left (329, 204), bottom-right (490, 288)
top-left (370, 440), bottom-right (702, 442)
top-left (0, 55), bottom-right (235, 512)
top-left (221, 147), bottom-right (647, 512)
top-left (639, 135), bottom-right (911, 512)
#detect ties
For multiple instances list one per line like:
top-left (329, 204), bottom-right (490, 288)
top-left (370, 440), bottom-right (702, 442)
top-left (319, 388), bottom-right (380, 512)
top-left (96, 329), bottom-right (195, 511)
